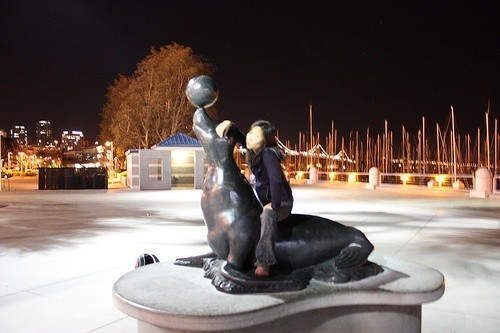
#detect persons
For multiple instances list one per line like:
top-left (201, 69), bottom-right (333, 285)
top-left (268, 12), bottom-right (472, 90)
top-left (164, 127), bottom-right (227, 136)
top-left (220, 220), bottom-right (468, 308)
top-left (215, 118), bottom-right (294, 277)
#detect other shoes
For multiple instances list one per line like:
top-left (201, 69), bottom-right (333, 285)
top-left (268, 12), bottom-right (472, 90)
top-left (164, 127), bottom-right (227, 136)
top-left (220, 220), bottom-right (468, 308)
top-left (255, 265), bottom-right (270, 275)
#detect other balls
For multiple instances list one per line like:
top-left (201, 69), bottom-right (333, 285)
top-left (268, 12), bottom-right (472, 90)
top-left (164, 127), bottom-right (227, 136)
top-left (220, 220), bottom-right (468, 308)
top-left (185, 75), bottom-right (219, 108)
